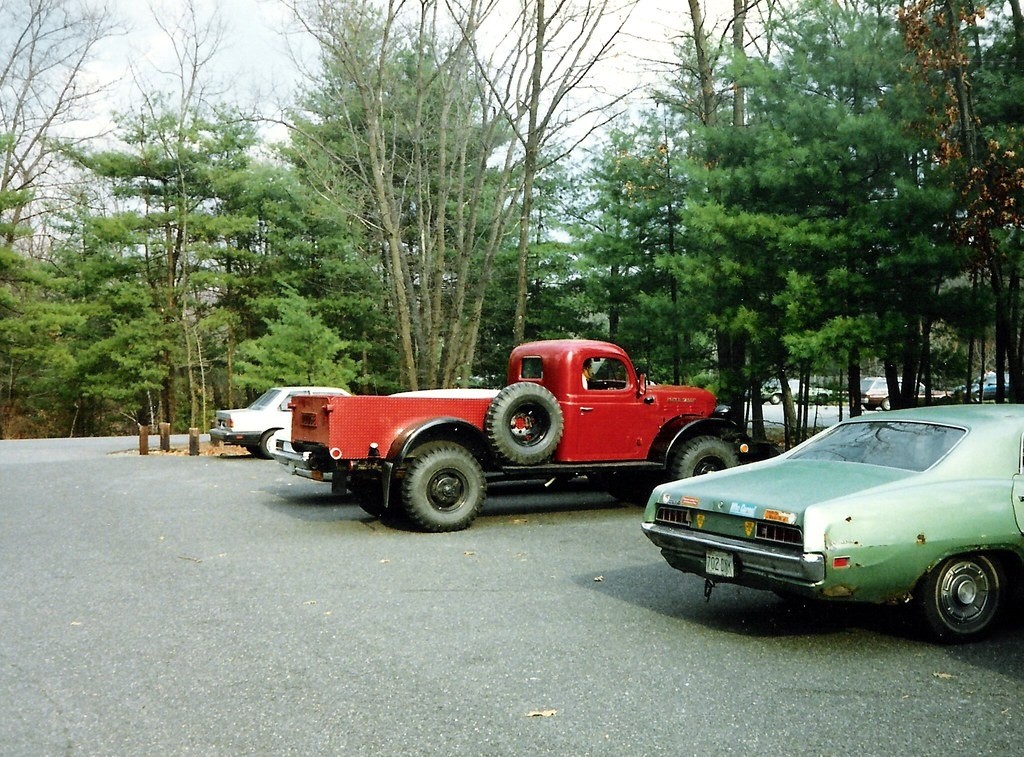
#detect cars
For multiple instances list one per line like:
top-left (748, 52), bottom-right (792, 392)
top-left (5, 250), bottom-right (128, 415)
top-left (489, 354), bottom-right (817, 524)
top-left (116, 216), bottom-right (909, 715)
top-left (954, 372), bottom-right (1009, 404)
top-left (210, 386), bottom-right (353, 461)
top-left (271, 389), bottom-right (501, 483)
top-left (761, 376), bottom-right (833, 406)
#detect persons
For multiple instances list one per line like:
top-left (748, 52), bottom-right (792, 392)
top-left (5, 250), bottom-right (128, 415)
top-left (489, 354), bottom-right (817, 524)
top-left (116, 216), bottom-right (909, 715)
top-left (581, 360), bottom-right (590, 390)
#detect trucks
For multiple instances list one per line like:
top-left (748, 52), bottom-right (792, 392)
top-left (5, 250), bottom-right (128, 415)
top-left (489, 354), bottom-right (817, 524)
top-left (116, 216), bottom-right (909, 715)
top-left (287, 339), bottom-right (781, 534)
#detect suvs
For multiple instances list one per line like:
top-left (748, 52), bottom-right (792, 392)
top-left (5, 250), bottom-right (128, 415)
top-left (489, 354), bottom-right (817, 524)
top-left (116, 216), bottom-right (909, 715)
top-left (862, 375), bottom-right (935, 412)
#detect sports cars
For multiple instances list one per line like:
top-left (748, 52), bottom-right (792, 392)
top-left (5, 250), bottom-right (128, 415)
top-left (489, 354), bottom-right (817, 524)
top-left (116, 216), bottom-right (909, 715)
top-left (640, 402), bottom-right (1024, 645)
